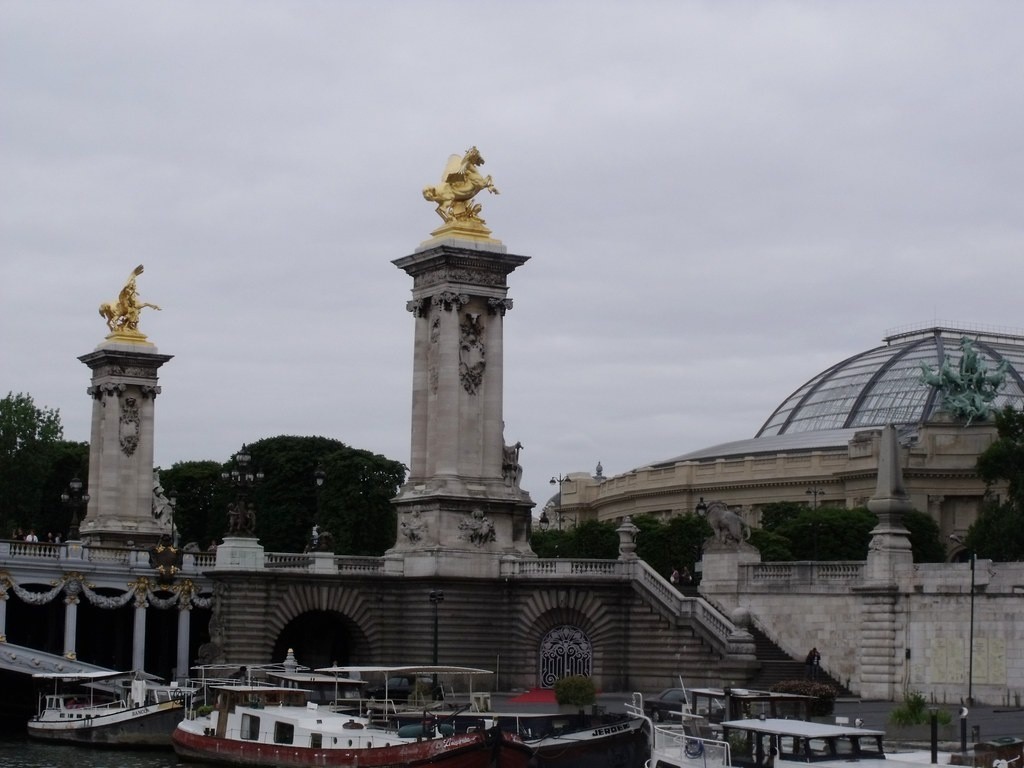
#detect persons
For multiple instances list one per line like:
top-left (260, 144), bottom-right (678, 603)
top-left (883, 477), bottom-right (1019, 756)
top-left (476, 518), bottom-right (491, 544)
top-left (228, 667), bottom-right (248, 685)
top-left (669, 566), bottom-right (690, 584)
top-left (12, 526), bottom-right (62, 557)
top-left (805, 648), bottom-right (819, 678)
top-left (502, 421), bottom-right (524, 487)
top-left (207, 540), bottom-right (217, 552)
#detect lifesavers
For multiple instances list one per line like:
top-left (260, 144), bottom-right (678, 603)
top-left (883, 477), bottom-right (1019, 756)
top-left (343, 722), bottom-right (364, 729)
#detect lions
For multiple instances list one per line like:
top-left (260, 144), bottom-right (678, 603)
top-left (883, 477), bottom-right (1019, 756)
top-left (707, 499), bottom-right (751, 546)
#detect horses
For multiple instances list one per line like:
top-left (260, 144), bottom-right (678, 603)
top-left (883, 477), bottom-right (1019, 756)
top-left (423, 146), bottom-right (494, 225)
top-left (98, 264), bottom-right (161, 330)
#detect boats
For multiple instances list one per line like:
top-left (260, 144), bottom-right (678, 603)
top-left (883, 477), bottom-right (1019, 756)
top-left (27, 645), bottom-right (979, 768)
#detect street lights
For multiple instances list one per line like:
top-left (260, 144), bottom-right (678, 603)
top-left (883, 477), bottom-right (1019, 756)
top-left (429, 589), bottom-right (445, 703)
top-left (313, 463), bottom-right (326, 549)
top-left (169, 484), bottom-right (178, 549)
top-left (949, 534), bottom-right (975, 698)
top-left (60, 478), bottom-right (91, 541)
top-left (549, 473), bottom-right (573, 530)
top-left (694, 497), bottom-right (708, 562)
top-left (218, 442), bottom-right (265, 538)
top-left (806, 484), bottom-right (826, 562)
